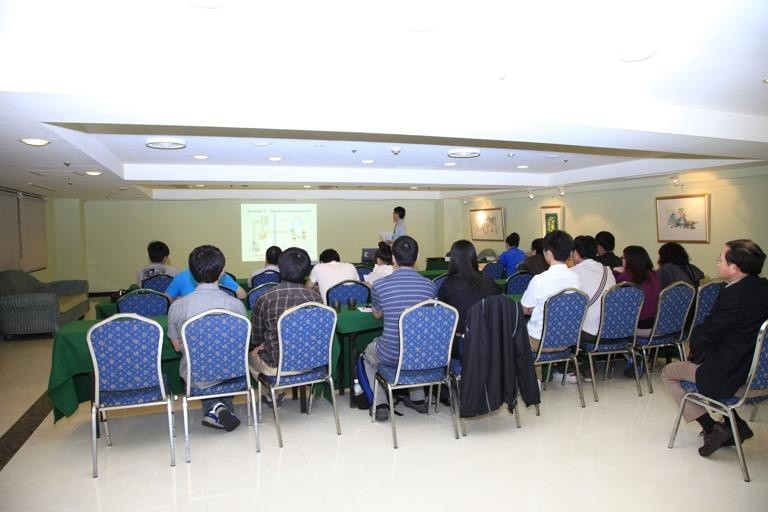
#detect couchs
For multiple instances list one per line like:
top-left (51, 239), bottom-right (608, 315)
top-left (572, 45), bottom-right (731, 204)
top-left (0, 269), bottom-right (90, 341)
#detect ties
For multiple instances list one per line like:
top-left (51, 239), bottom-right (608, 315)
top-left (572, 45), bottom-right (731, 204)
top-left (393, 223), bottom-right (398, 235)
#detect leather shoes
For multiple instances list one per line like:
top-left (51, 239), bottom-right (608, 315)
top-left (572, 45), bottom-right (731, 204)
top-left (404, 396), bottom-right (427, 413)
top-left (724, 420), bottom-right (754, 448)
top-left (699, 421), bottom-right (731, 457)
top-left (369, 404), bottom-right (389, 420)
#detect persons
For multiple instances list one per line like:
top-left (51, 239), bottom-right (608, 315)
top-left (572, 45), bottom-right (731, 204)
top-left (166, 244), bottom-right (247, 432)
top-left (550, 215), bottom-right (557, 233)
top-left (660, 239), bottom-right (768, 458)
top-left (497, 230), bottom-right (622, 279)
top-left (518, 230), bottom-right (582, 394)
top-left (668, 207), bottom-right (699, 229)
top-left (164, 266), bottom-right (246, 305)
top-left (612, 245), bottom-right (662, 379)
top-left (438, 239), bottom-right (502, 407)
top-left (384, 206), bottom-right (407, 248)
top-left (553, 234), bottom-right (617, 384)
top-left (656, 242), bottom-right (706, 330)
top-left (135, 239), bottom-right (177, 289)
top-left (362, 235), bottom-right (436, 423)
top-left (247, 247), bottom-right (312, 411)
top-left (246, 246), bottom-right (283, 289)
top-left (305, 248), bottom-right (361, 307)
top-left (569, 235), bottom-right (617, 383)
top-left (363, 240), bottom-right (398, 287)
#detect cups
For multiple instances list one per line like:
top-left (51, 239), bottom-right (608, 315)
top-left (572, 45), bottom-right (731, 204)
top-left (347, 296), bottom-right (357, 310)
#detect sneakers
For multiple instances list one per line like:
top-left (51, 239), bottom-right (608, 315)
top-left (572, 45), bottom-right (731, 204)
top-left (554, 370), bottom-right (595, 384)
top-left (261, 390), bottom-right (286, 410)
top-left (625, 354), bottom-right (644, 378)
top-left (200, 402), bottom-right (241, 432)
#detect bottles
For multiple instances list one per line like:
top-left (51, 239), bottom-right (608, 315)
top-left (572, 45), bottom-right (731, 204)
top-left (352, 379), bottom-right (362, 395)
top-left (331, 297), bottom-right (340, 312)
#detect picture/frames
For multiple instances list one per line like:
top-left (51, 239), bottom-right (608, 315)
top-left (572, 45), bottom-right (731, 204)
top-left (469, 206), bottom-right (506, 243)
top-left (655, 192), bottom-right (712, 244)
top-left (539, 204), bottom-right (566, 238)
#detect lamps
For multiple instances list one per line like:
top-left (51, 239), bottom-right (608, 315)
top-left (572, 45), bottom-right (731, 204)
top-left (447, 147), bottom-right (482, 159)
top-left (144, 137), bottom-right (186, 150)
